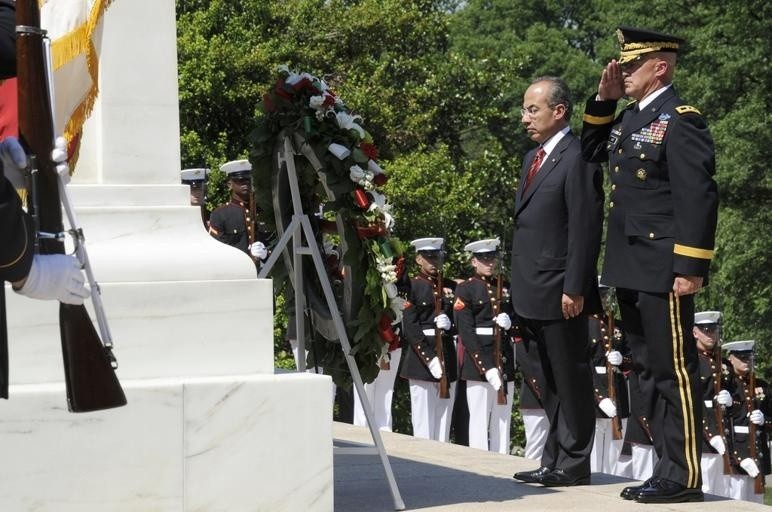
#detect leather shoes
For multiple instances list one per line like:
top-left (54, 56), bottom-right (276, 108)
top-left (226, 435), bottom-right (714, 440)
top-left (514, 466), bottom-right (590, 485)
top-left (621, 475), bottom-right (704, 503)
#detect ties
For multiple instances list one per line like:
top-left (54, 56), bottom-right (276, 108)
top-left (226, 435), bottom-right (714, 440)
top-left (524, 148), bottom-right (545, 191)
top-left (621, 102), bottom-right (639, 131)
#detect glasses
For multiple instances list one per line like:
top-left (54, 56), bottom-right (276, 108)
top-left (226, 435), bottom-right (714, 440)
top-left (520, 102), bottom-right (568, 116)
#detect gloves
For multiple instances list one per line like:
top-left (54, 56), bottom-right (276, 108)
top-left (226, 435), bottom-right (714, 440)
top-left (717, 390), bottom-right (733, 407)
top-left (12, 253), bottom-right (90, 305)
top-left (434, 314), bottom-right (451, 331)
top-left (492, 313), bottom-right (511, 330)
top-left (485, 366), bottom-right (503, 391)
top-left (605, 351), bottom-right (623, 366)
top-left (709, 435), bottom-right (726, 456)
top-left (740, 457), bottom-right (760, 478)
top-left (248, 242), bottom-right (267, 260)
top-left (427, 356), bottom-right (444, 379)
top-left (0, 137), bottom-right (70, 190)
top-left (598, 398), bottom-right (617, 417)
top-left (747, 410), bottom-right (765, 426)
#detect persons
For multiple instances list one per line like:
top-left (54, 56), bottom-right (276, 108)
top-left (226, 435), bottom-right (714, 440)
top-left (694, 311), bottom-right (771, 505)
top-left (0, 0), bottom-right (91, 399)
top-left (181, 167), bottom-right (206, 204)
top-left (353, 325), bottom-right (403, 431)
top-left (512, 76), bottom-right (605, 488)
top-left (579, 26), bottom-right (720, 504)
top-left (209, 159), bottom-right (267, 273)
top-left (452, 239), bottom-right (515, 456)
top-left (513, 338), bottom-right (551, 460)
top-left (284, 310), bottom-right (324, 375)
top-left (399, 237), bottom-right (460, 442)
top-left (590, 274), bottom-right (660, 480)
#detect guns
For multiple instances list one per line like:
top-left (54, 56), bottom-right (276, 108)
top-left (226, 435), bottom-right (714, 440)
top-left (716, 343), bottom-right (733, 475)
top-left (14, 0), bottom-right (127, 413)
top-left (248, 191), bottom-right (262, 270)
top-left (749, 356), bottom-right (765, 494)
top-left (495, 275), bottom-right (507, 404)
top-left (433, 270), bottom-right (450, 398)
top-left (608, 312), bottom-right (623, 441)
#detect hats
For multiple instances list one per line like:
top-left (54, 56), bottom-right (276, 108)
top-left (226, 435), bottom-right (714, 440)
top-left (721, 340), bottom-right (755, 357)
top-left (411, 238), bottom-right (444, 256)
top-left (181, 168), bottom-right (206, 189)
top-left (694, 311), bottom-right (720, 331)
top-left (464, 239), bottom-right (501, 260)
top-left (616, 26), bottom-right (686, 64)
top-left (220, 160), bottom-right (252, 180)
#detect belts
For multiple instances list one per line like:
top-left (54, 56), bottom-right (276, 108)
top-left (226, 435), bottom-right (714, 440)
top-left (596, 366), bottom-right (623, 374)
top-left (734, 426), bottom-right (749, 433)
top-left (476, 328), bottom-right (501, 336)
top-left (423, 329), bottom-right (445, 336)
top-left (704, 400), bottom-right (726, 408)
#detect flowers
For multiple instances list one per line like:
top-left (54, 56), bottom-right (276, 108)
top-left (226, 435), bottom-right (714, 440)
top-left (244, 62), bottom-right (415, 401)
